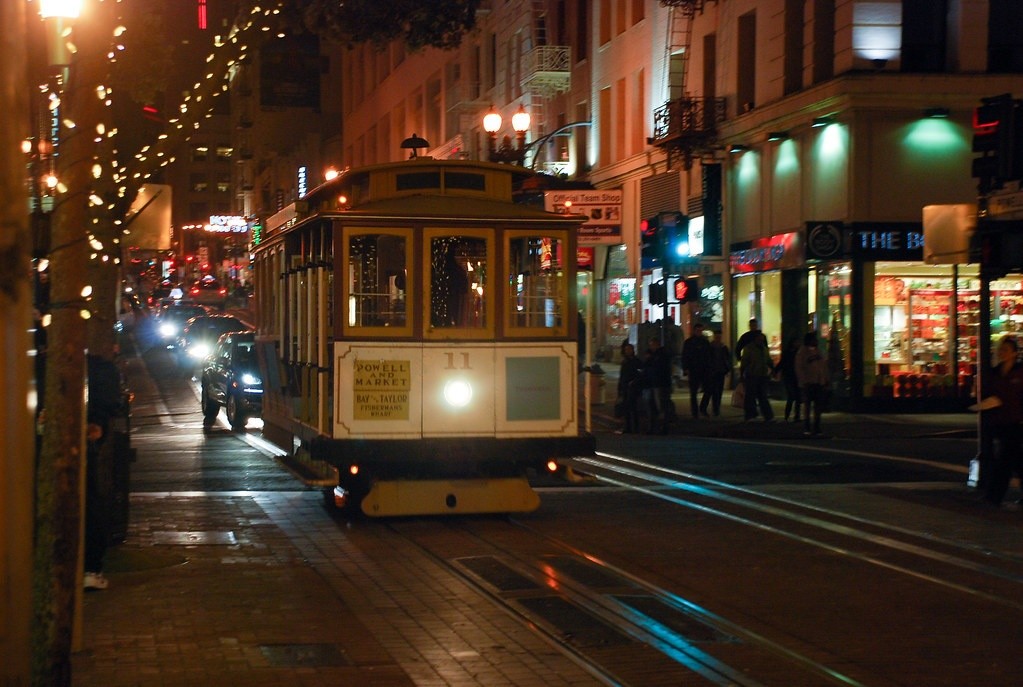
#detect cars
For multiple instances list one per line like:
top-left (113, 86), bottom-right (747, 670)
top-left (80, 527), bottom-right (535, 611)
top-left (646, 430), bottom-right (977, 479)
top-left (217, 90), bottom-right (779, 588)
top-left (174, 312), bottom-right (249, 380)
top-left (199, 331), bottom-right (263, 428)
top-left (130, 257), bottom-right (218, 350)
top-left (219, 286), bottom-right (250, 312)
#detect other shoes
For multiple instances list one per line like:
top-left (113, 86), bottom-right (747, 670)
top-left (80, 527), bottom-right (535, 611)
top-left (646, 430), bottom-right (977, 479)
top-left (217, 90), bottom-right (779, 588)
top-left (801, 427), bottom-right (812, 434)
top-left (691, 408), bottom-right (697, 419)
top-left (699, 407), bottom-right (709, 417)
top-left (82, 570), bottom-right (107, 589)
top-left (814, 430), bottom-right (825, 436)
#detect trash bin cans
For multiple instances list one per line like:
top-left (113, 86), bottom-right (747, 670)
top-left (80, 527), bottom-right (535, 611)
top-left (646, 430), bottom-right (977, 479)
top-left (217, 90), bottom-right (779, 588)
top-left (35, 353), bottom-right (130, 546)
top-left (591, 364), bottom-right (607, 404)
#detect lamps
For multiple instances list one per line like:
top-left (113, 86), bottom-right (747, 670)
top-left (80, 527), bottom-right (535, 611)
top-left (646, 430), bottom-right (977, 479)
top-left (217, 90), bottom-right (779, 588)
top-left (871, 59), bottom-right (888, 73)
top-left (766, 131), bottom-right (788, 142)
top-left (729, 144), bottom-right (748, 153)
top-left (811, 117), bottom-right (834, 127)
top-left (922, 107), bottom-right (947, 118)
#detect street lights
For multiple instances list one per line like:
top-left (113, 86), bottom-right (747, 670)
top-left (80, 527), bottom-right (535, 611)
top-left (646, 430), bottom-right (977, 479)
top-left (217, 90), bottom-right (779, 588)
top-left (482, 97), bottom-right (531, 168)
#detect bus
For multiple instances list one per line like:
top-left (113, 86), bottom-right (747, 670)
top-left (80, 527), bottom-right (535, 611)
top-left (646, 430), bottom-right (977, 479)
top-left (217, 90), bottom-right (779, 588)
top-left (253, 154), bottom-right (595, 522)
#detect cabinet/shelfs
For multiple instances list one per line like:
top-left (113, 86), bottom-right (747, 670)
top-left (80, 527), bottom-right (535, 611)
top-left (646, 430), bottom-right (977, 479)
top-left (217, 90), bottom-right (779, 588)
top-left (907, 288), bottom-right (1023, 366)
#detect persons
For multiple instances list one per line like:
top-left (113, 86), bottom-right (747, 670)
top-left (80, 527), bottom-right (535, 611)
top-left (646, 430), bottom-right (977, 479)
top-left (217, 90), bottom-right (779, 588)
top-left (767, 335), bottom-right (802, 425)
top-left (681, 324), bottom-right (710, 420)
top-left (979, 337), bottom-right (1023, 510)
top-left (664, 316), bottom-right (683, 387)
top-left (794, 333), bottom-right (829, 436)
top-left (699, 328), bottom-right (733, 417)
top-left (740, 334), bottom-right (776, 422)
top-left (735, 318), bottom-right (768, 361)
top-left (34, 352), bottom-right (121, 589)
top-left (645, 338), bottom-right (672, 438)
top-left (616, 344), bottom-right (646, 433)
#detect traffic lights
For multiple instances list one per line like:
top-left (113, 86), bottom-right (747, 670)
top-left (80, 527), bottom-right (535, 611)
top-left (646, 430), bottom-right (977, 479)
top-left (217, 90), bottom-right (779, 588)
top-left (968, 103), bottom-right (1012, 190)
top-left (639, 215), bottom-right (659, 259)
top-left (673, 278), bottom-right (698, 304)
top-left (675, 215), bottom-right (691, 260)
top-left (968, 228), bottom-right (1003, 265)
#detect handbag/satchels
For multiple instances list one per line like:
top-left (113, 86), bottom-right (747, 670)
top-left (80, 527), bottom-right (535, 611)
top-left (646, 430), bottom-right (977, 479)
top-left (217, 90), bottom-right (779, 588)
top-left (731, 382), bottom-right (745, 406)
top-left (613, 396), bottom-right (625, 417)
